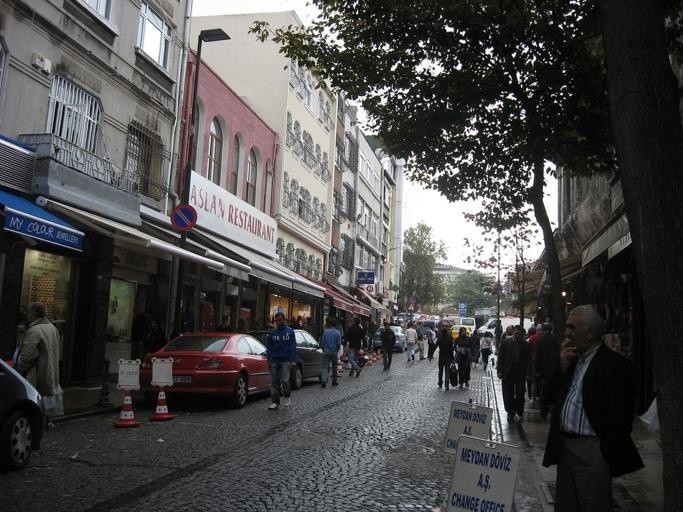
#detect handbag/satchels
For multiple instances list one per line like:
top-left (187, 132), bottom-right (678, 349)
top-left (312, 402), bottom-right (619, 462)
top-left (448, 363), bottom-right (458, 387)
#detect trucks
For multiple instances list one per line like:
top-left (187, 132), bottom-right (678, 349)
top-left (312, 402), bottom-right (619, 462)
top-left (477, 317), bottom-right (530, 336)
top-left (396, 312), bottom-right (440, 327)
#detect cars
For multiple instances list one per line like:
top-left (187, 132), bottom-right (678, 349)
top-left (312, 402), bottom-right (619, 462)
top-left (0, 357), bottom-right (48, 469)
top-left (248, 327), bottom-right (329, 390)
top-left (447, 324), bottom-right (473, 339)
top-left (142, 330), bottom-right (271, 410)
top-left (373, 325), bottom-right (405, 354)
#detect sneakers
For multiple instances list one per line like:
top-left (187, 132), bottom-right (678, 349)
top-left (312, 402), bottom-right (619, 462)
top-left (511, 416), bottom-right (523, 423)
top-left (284, 397), bottom-right (290, 407)
top-left (268, 402), bottom-right (279, 409)
top-left (507, 413), bottom-right (514, 421)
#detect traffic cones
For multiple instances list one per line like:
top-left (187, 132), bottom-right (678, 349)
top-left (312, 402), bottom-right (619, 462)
top-left (376, 349), bottom-right (381, 360)
top-left (366, 354), bottom-right (372, 366)
top-left (371, 353), bottom-right (377, 365)
top-left (345, 359), bottom-right (351, 369)
top-left (144, 387), bottom-right (175, 422)
top-left (114, 392), bottom-right (140, 428)
top-left (336, 357), bottom-right (344, 377)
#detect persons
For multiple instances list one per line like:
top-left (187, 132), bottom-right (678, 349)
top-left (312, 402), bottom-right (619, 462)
top-left (405, 322), bottom-right (436, 361)
top-left (361, 320), bottom-right (376, 348)
top-left (344, 318), bottom-right (366, 378)
top-left (381, 321), bottom-right (396, 371)
top-left (265, 312), bottom-right (297, 409)
top-left (378, 319), bottom-right (401, 326)
top-left (293, 315), bottom-right (312, 333)
top-left (10, 300), bottom-right (59, 451)
top-left (497, 322), bottom-right (559, 423)
top-left (321, 318), bottom-right (341, 387)
top-left (542, 306), bottom-right (646, 512)
top-left (433, 327), bottom-right (492, 390)
top-left (216, 314), bottom-right (248, 334)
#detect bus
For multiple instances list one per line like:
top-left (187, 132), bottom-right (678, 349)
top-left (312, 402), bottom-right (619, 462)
top-left (441, 317), bottom-right (476, 333)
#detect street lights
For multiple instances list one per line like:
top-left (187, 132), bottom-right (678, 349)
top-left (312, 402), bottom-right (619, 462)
top-left (165, 26), bottom-right (232, 336)
